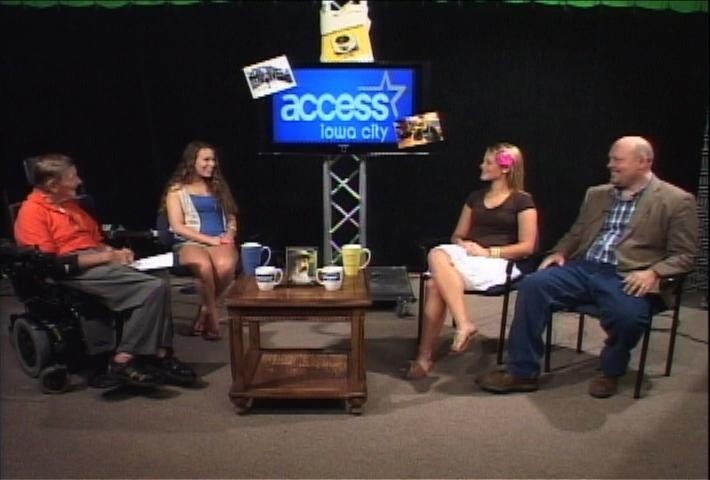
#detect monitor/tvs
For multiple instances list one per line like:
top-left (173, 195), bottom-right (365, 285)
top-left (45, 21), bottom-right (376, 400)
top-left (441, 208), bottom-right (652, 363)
top-left (266, 62), bottom-right (421, 151)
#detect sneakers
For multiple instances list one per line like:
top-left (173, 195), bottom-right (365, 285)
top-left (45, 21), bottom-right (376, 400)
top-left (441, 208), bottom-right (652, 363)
top-left (588, 371), bottom-right (618, 398)
top-left (105, 357), bottom-right (160, 387)
top-left (483, 369), bottom-right (538, 395)
top-left (150, 354), bottom-right (196, 387)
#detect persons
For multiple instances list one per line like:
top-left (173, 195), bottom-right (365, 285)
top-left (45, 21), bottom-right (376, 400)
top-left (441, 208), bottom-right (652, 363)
top-left (411, 114), bottom-right (442, 145)
top-left (11, 151), bottom-right (204, 392)
top-left (471, 135), bottom-right (703, 399)
top-left (399, 140), bottom-right (540, 381)
top-left (262, 71), bottom-right (272, 89)
top-left (160, 139), bottom-right (240, 341)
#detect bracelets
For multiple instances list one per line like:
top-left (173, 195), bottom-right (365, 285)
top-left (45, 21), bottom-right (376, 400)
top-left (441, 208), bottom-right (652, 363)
top-left (485, 245), bottom-right (504, 260)
top-left (227, 227), bottom-right (239, 231)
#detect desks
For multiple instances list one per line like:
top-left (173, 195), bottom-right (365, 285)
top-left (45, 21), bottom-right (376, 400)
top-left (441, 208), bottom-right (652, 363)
top-left (214, 267), bottom-right (374, 418)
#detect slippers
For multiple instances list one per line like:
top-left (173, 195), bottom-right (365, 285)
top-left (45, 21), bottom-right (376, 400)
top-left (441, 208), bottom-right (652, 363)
top-left (451, 326), bottom-right (480, 356)
top-left (405, 354), bottom-right (443, 380)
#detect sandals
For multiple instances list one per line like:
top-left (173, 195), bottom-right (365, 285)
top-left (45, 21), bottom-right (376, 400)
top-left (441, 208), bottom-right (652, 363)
top-left (192, 305), bottom-right (223, 341)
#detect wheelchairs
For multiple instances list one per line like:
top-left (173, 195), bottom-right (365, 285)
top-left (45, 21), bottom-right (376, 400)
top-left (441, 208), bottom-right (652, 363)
top-left (0, 159), bottom-right (172, 397)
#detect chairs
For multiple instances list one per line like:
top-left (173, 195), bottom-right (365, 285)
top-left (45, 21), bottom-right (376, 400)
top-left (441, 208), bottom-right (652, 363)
top-left (415, 243), bottom-right (546, 365)
top-left (527, 251), bottom-right (692, 400)
top-left (156, 206), bottom-right (197, 277)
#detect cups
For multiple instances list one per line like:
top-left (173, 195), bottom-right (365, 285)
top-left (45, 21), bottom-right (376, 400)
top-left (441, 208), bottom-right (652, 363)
top-left (342, 244), bottom-right (371, 277)
top-left (240, 242), bottom-right (271, 278)
top-left (315, 265), bottom-right (343, 293)
top-left (254, 267), bottom-right (283, 292)
